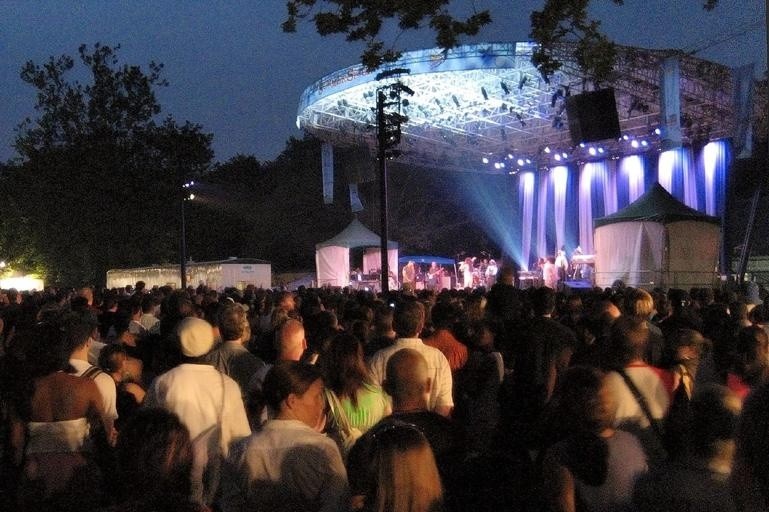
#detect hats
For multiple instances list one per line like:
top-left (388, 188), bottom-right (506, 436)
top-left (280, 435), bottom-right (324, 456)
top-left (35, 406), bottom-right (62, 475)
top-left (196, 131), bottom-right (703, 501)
top-left (177, 316), bottom-right (214, 358)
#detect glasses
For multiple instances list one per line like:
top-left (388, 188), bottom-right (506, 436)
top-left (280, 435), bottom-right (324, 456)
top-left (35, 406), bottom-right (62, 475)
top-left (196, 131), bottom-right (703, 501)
top-left (360, 422), bottom-right (425, 445)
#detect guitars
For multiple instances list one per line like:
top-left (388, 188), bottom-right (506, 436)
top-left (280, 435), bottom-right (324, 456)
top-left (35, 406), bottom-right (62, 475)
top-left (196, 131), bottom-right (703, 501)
top-left (425, 267), bottom-right (444, 281)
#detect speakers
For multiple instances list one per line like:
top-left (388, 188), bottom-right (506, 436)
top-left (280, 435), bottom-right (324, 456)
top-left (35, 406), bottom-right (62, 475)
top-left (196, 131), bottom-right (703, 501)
top-left (564, 87), bottom-right (621, 145)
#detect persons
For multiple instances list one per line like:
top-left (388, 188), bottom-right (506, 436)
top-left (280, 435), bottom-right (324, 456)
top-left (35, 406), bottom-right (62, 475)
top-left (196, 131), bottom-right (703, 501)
top-left (401, 251), bottom-right (497, 291)
top-left (350, 264), bottom-right (394, 282)
top-left (532, 246), bottom-right (585, 287)
top-left (1, 281), bottom-right (769, 512)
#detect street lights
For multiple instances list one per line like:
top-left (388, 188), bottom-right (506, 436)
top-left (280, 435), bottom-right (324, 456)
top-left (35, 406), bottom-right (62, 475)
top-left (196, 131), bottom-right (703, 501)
top-left (175, 179), bottom-right (200, 291)
top-left (375, 86), bottom-right (408, 293)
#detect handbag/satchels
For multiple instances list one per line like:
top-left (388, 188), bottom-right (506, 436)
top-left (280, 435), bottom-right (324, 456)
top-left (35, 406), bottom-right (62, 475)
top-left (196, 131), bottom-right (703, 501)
top-left (333, 427), bottom-right (362, 460)
